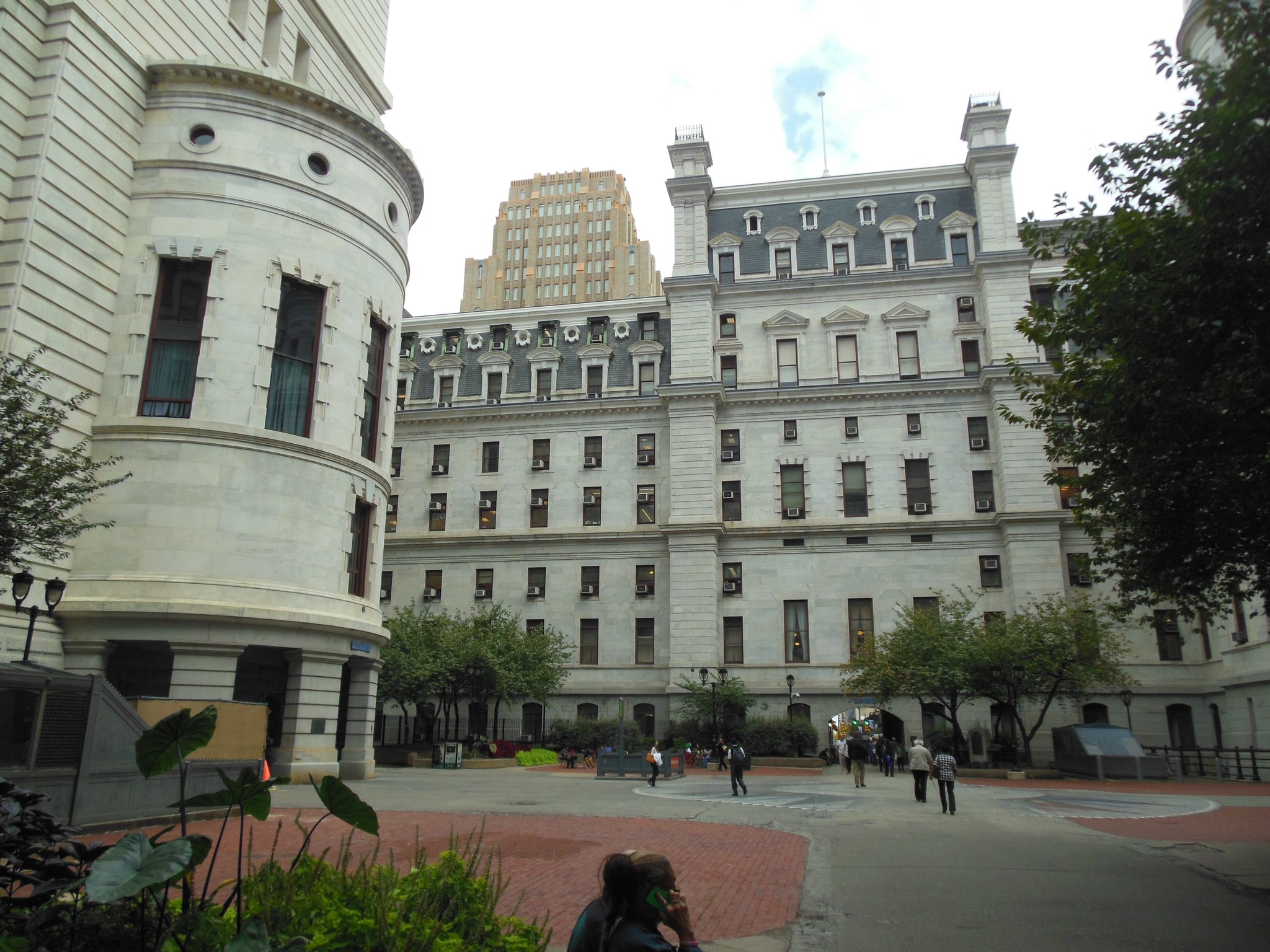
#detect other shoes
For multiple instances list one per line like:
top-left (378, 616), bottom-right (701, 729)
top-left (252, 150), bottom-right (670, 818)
top-left (942, 811), bottom-right (946, 813)
top-left (856, 785), bottom-right (859, 788)
top-left (885, 775), bottom-right (888, 776)
top-left (861, 784), bottom-right (866, 787)
top-left (586, 766), bottom-right (595, 769)
top-left (922, 800), bottom-right (926, 803)
top-left (950, 810), bottom-right (954, 815)
top-left (847, 772), bottom-right (850, 775)
top-left (891, 776), bottom-right (893, 777)
top-left (571, 766), bottom-right (575, 768)
top-left (732, 793), bottom-right (738, 796)
top-left (742, 786), bottom-right (747, 795)
top-left (917, 799), bottom-right (920, 801)
top-left (647, 781), bottom-right (657, 788)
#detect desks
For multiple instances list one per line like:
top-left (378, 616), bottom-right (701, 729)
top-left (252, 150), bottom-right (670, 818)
top-left (692, 753), bottom-right (711, 767)
top-left (570, 753), bottom-right (586, 769)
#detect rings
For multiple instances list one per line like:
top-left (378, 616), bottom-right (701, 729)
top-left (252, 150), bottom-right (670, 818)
top-left (671, 900), bottom-right (679, 904)
top-left (663, 900), bottom-right (668, 907)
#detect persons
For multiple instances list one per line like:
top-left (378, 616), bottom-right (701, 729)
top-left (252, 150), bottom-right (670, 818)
top-left (566, 850), bottom-right (704, 952)
top-left (560, 730), bottom-right (959, 815)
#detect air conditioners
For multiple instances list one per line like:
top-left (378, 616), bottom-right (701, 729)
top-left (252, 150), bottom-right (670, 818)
top-left (400, 334), bottom-right (602, 358)
top-left (386, 493), bottom-right (648, 512)
top-left (779, 264), bottom-right (906, 279)
top-left (723, 583), bottom-right (736, 592)
top-left (380, 585), bottom-right (647, 597)
top-left (915, 501), bottom-right (998, 569)
top-left (723, 451), bottom-right (733, 498)
top-left (785, 424), bottom-right (920, 438)
top-left (1079, 575), bottom-right (1090, 582)
top-left (1068, 497), bottom-right (1082, 506)
top-left (532, 455), bottom-right (649, 469)
top-left (960, 298), bottom-right (972, 309)
top-left (970, 439), bottom-right (984, 450)
top-left (431, 465), bottom-right (443, 473)
top-left (396, 393), bottom-right (598, 411)
top-left (788, 508), bottom-right (801, 519)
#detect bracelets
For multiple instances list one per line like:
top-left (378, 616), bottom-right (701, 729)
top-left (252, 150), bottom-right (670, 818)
top-left (679, 940), bottom-right (699, 946)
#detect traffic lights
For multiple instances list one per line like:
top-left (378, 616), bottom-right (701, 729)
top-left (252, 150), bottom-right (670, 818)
top-left (864, 723), bottom-right (869, 732)
top-left (853, 718), bottom-right (857, 725)
top-left (840, 722), bottom-right (845, 733)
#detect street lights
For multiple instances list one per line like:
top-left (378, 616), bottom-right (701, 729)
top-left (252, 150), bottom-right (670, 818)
top-left (1119, 689), bottom-right (1133, 732)
top-left (12, 569), bottom-right (67, 662)
top-left (698, 667), bottom-right (728, 764)
top-left (786, 674), bottom-right (800, 725)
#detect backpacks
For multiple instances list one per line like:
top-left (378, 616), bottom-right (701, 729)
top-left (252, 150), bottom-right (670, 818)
top-left (730, 747), bottom-right (745, 766)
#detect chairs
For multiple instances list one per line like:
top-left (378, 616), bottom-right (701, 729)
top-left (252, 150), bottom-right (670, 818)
top-left (558, 751), bottom-right (597, 769)
top-left (684, 752), bottom-right (719, 769)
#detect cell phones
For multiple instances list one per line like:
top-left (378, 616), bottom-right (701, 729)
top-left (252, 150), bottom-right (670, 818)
top-left (646, 885), bottom-right (672, 917)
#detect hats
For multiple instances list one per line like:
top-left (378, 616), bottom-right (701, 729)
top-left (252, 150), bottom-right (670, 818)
top-left (879, 733), bottom-right (883, 737)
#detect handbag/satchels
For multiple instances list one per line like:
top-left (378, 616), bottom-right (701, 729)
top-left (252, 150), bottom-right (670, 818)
top-left (872, 748), bottom-right (876, 754)
top-left (646, 752), bottom-right (655, 763)
top-left (931, 755), bottom-right (940, 778)
